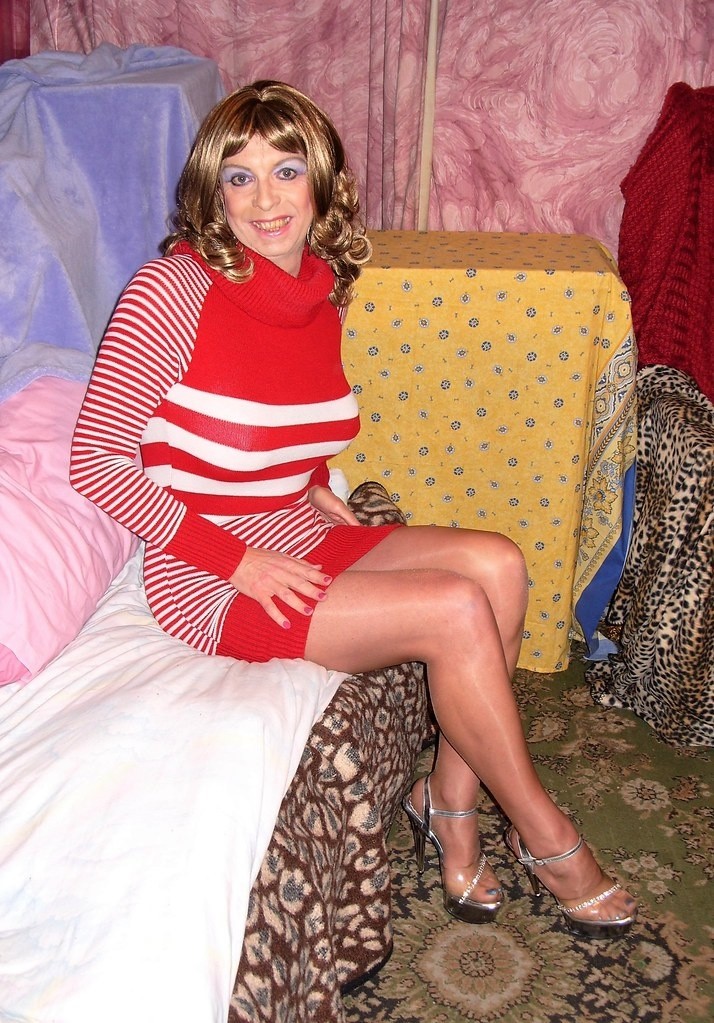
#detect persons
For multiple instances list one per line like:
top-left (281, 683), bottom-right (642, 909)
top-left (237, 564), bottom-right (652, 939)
top-left (69, 78), bottom-right (638, 939)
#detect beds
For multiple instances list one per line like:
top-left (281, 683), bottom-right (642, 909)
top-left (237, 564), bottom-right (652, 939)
top-left (0, 342), bottom-right (434, 1023)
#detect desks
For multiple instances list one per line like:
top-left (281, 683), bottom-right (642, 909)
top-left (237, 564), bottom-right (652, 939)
top-left (321, 230), bottom-right (640, 677)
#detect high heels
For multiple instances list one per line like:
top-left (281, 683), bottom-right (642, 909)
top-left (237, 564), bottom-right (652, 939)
top-left (504, 824), bottom-right (639, 938)
top-left (402, 772), bottom-right (502, 924)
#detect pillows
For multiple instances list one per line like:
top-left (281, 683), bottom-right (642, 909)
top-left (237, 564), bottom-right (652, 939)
top-left (0, 340), bottom-right (147, 687)
